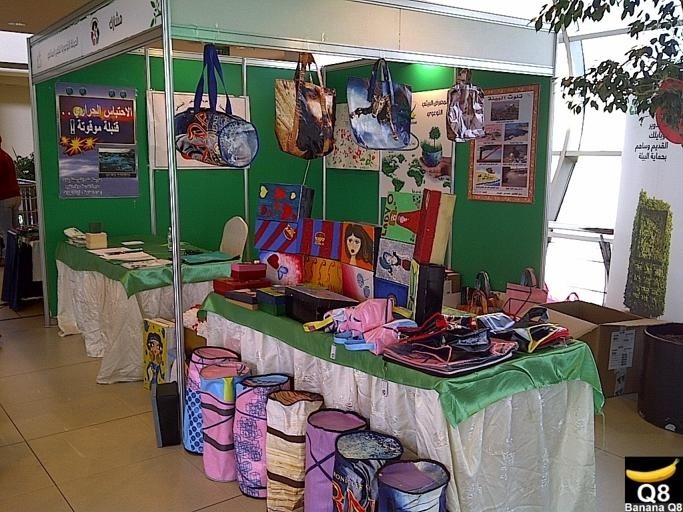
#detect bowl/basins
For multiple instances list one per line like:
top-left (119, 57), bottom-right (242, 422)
top-left (420, 149), bottom-right (442, 167)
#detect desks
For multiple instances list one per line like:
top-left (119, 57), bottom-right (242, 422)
top-left (54, 229), bottom-right (232, 378)
top-left (205, 294), bottom-right (592, 512)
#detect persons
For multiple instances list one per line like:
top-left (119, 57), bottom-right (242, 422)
top-left (1, 138), bottom-right (21, 268)
top-left (301, 80), bottom-right (334, 135)
top-left (342, 225), bottom-right (371, 269)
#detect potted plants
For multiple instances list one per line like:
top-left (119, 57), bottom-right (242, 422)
top-left (524, 0), bottom-right (683, 436)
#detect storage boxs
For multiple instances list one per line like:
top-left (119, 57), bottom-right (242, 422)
top-left (516, 298), bottom-right (674, 402)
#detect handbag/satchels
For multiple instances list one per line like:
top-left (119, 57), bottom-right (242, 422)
top-left (174, 44), bottom-right (259, 167)
top-left (375, 459), bottom-right (450, 512)
top-left (346, 58), bottom-right (411, 150)
top-left (183, 347), bottom-right (240, 454)
top-left (264, 391), bottom-right (323, 512)
top-left (305, 410), bottom-right (366, 512)
top-left (303, 267), bottom-right (574, 379)
top-left (274, 54), bottom-right (336, 159)
top-left (198, 363), bottom-right (251, 481)
top-left (332, 431), bottom-right (403, 512)
top-left (446, 67), bottom-right (486, 143)
top-left (234, 373), bottom-right (292, 499)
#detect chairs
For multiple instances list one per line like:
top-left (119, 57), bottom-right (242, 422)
top-left (219, 215), bottom-right (249, 262)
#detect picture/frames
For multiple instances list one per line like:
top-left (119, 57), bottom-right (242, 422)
top-left (467, 83), bottom-right (541, 205)
